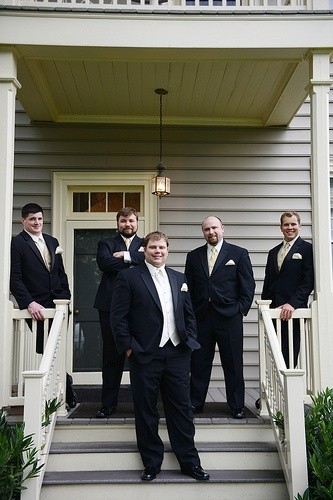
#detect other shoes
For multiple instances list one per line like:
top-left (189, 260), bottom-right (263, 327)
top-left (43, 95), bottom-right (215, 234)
top-left (256, 398), bottom-right (261, 409)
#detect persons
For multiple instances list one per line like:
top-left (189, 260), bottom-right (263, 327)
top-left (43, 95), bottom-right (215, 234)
top-left (184, 216), bottom-right (255, 417)
top-left (94, 206), bottom-right (145, 417)
top-left (255, 212), bottom-right (314, 409)
top-left (111, 231), bottom-right (210, 480)
top-left (11, 202), bottom-right (77, 407)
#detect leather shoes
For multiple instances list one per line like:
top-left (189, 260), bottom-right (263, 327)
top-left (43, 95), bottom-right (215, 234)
top-left (191, 405), bottom-right (201, 414)
top-left (95, 407), bottom-right (114, 417)
top-left (179, 467), bottom-right (209, 480)
top-left (66, 377), bottom-right (76, 408)
top-left (141, 468), bottom-right (160, 480)
top-left (232, 408), bottom-right (245, 418)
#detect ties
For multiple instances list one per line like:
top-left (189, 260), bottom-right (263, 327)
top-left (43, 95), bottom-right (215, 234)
top-left (207, 247), bottom-right (217, 277)
top-left (125, 239), bottom-right (130, 251)
top-left (277, 243), bottom-right (289, 271)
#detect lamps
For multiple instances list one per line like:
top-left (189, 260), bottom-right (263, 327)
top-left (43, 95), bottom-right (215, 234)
top-left (152, 89), bottom-right (171, 199)
